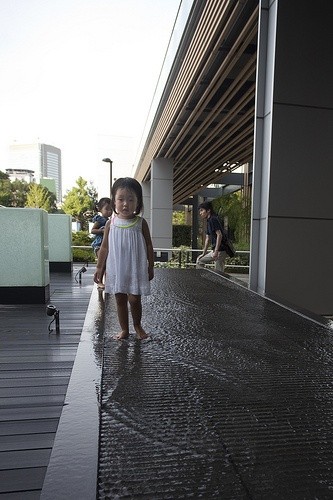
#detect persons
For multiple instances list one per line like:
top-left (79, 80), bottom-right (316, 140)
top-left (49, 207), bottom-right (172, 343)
top-left (91, 197), bottom-right (114, 289)
top-left (196, 203), bottom-right (227, 273)
top-left (94, 177), bottom-right (154, 341)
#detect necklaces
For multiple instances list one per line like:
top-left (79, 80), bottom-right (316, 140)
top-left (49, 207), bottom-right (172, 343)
top-left (112, 216), bottom-right (139, 228)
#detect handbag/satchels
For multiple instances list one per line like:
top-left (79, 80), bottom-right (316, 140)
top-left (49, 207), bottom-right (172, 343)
top-left (225, 241), bottom-right (236, 257)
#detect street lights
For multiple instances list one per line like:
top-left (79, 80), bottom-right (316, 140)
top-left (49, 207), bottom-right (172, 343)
top-left (102, 158), bottom-right (112, 200)
